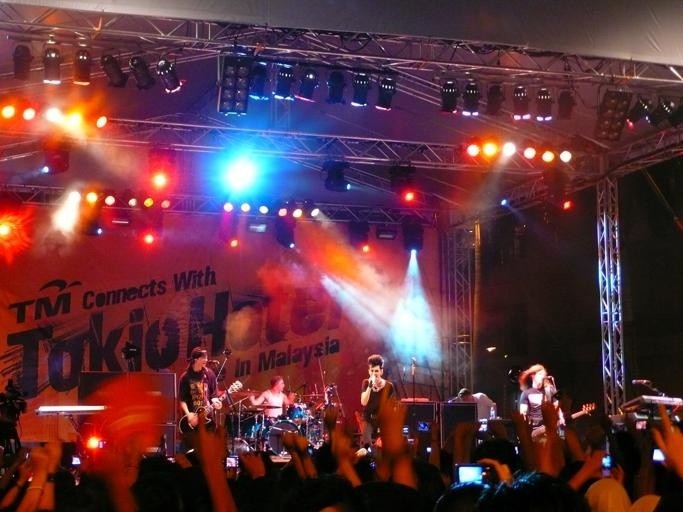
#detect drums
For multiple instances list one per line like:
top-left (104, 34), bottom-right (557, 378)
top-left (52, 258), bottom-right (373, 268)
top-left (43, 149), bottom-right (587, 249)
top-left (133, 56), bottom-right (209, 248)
top-left (265, 420), bottom-right (301, 455)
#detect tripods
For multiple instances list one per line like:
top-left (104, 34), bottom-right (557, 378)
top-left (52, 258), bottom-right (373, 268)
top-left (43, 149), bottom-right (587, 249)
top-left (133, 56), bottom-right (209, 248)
top-left (0, 430), bottom-right (22, 471)
top-left (229, 396), bottom-right (344, 454)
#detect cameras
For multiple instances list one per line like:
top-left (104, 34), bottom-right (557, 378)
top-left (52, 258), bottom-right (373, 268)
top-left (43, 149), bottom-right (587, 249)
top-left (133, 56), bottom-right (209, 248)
top-left (225, 456), bottom-right (241, 469)
top-left (455, 463), bottom-right (493, 485)
top-left (71, 456), bottom-right (81, 467)
top-left (601, 455), bottom-right (611, 470)
top-left (650, 447), bottom-right (665, 463)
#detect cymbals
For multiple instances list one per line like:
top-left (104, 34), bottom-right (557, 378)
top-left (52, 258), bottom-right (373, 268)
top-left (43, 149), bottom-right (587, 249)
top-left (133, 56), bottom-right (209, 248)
top-left (301, 394), bottom-right (325, 396)
top-left (229, 391), bottom-right (264, 395)
top-left (249, 405), bottom-right (285, 408)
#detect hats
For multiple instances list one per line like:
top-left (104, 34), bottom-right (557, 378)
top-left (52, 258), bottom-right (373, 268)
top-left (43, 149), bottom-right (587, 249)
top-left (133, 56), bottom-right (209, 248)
top-left (186, 347), bottom-right (207, 361)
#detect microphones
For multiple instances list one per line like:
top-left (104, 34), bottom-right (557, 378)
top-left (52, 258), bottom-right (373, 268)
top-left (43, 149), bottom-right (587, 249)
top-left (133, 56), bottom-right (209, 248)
top-left (209, 360), bottom-right (219, 364)
top-left (542, 376), bottom-right (551, 380)
top-left (369, 381), bottom-right (373, 388)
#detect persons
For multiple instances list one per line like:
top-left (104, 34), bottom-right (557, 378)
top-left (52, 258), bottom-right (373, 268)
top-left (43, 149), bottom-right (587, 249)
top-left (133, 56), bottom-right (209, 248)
top-left (2, 346), bottom-right (683, 512)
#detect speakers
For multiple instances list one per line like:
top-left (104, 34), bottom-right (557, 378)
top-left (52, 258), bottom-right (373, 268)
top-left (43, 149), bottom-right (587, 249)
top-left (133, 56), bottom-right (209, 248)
top-left (439, 402), bottom-right (479, 474)
top-left (371, 402), bottom-right (441, 473)
top-left (76, 371), bottom-right (176, 459)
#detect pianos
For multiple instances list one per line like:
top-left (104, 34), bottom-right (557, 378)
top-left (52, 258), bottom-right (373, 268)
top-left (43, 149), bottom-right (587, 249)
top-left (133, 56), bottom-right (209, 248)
top-left (35, 405), bottom-right (111, 416)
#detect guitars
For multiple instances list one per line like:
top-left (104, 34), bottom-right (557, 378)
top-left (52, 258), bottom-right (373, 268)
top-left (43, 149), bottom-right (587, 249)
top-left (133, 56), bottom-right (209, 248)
top-left (531, 402), bottom-right (595, 444)
top-left (179, 381), bottom-right (243, 434)
